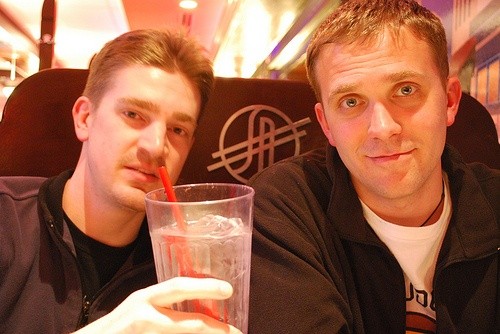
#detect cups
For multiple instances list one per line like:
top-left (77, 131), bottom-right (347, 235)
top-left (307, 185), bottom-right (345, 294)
top-left (143, 182), bottom-right (256, 334)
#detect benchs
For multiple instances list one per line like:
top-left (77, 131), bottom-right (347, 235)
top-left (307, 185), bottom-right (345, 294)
top-left (1, 68), bottom-right (497, 199)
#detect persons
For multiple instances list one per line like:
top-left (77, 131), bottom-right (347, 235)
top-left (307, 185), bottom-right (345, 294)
top-left (0, 29), bottom-right (242, 334)
top-left (247, 0), bottom-right (500, 334)
